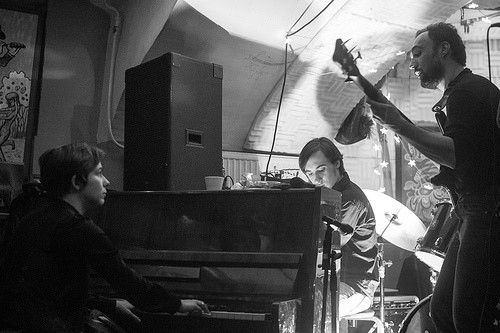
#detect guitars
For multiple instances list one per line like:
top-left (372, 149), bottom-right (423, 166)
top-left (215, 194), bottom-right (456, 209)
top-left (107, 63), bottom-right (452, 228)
top-left (331, 38), bottom-right (461, 211)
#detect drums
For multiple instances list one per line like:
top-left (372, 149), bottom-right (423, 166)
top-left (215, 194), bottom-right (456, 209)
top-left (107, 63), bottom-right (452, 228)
top-left (398, 294), bottom-right (437, 333)
top-left (413, 200), bottom-right (462, 274)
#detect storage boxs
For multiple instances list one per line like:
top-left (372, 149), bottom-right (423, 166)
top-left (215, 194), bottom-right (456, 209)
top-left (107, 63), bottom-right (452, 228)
top-left (348, 295), bottom-right (421, 333)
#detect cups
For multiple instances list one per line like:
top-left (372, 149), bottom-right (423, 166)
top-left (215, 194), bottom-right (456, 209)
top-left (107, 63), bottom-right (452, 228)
top-left (205, 176), bottom-right (224, 190)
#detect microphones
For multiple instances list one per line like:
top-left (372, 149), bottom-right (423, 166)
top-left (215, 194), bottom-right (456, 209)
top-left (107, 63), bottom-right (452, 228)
top-left (322, 216), bottom-right (354, 235)
top-left (290, 177), bottom-right (316, 188)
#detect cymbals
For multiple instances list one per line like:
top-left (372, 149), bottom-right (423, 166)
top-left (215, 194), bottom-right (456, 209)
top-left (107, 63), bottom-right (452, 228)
top-left (361, 188), bottom-right (428, 252)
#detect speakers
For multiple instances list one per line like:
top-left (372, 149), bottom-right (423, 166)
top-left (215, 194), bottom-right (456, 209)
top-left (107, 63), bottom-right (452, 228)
top-left (124, 51), bottom-right (224, 192)
top-left (346, 295), bottom-right (421, 333)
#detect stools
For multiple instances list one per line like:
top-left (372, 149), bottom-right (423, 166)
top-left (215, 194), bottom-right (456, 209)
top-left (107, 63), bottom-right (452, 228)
top-left (343, 309), bottom-right (384, 333)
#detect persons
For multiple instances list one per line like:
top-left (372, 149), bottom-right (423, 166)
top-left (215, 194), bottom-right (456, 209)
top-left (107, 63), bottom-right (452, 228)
top-left (298, 137), bottom-right (380, 324)
top-left (0, 143), bottom-right (210, 333)
top-left (364, 22), bottom-right (500, 333)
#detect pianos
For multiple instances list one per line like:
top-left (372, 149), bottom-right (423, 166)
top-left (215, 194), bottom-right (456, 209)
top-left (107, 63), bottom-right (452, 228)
top-left (94, 185), bottom-right (343, 333)
